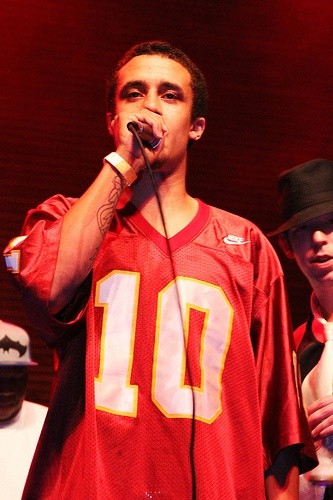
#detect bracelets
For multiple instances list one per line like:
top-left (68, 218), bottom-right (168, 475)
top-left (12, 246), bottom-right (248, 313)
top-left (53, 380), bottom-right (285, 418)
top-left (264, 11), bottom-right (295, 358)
top-left (101, 152), bottom-right (137, 186)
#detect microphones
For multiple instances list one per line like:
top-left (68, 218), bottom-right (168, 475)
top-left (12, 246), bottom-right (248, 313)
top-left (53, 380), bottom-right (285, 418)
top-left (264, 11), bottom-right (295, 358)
top-left (126, 120), bottom-right (163, 150)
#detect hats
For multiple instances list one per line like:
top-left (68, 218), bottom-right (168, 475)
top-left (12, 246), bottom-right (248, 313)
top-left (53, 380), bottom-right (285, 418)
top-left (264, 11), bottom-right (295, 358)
top-left (264, 158), bottom-right (333, 238)
top-left (0, 319), bottom-right (38, 366)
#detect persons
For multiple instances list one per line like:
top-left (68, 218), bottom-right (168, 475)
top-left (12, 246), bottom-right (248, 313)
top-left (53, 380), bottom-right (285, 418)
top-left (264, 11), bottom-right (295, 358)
top-left (3, 39), bottom-right (321, 500)
top-left (0, 320), bottom-right (49, 500)
top-left (264, 156), bottom-right (332, 500)
